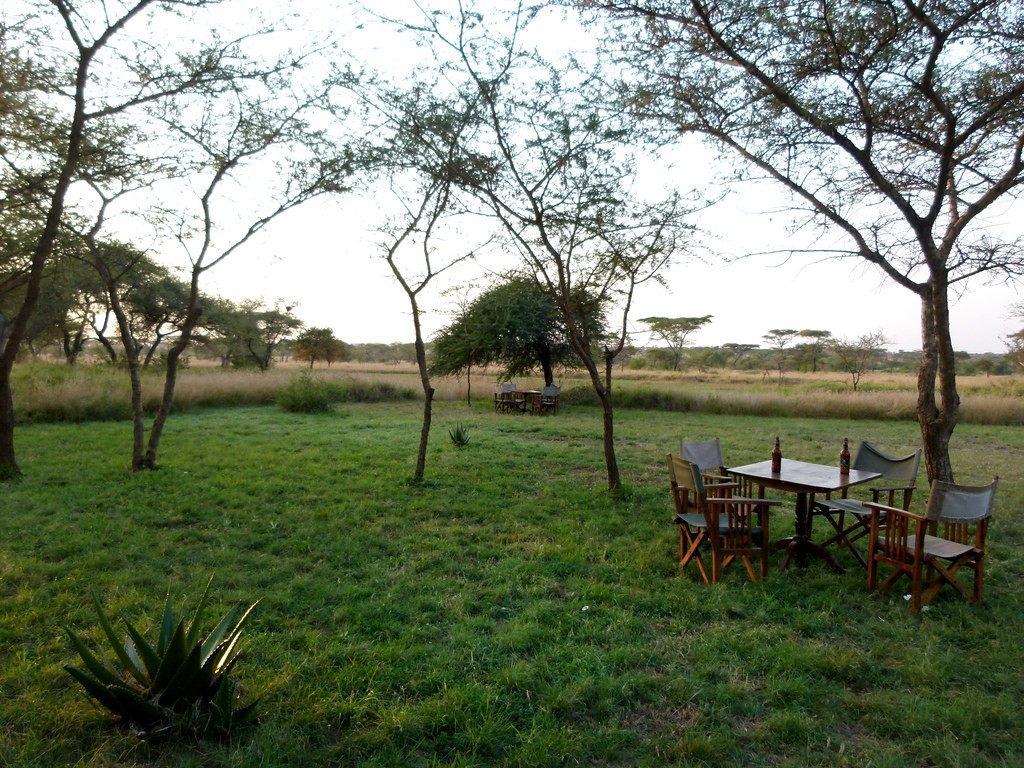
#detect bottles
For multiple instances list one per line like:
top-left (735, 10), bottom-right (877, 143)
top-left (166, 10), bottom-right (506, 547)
top-left (771, 436), bottom-right (782, 473)
top-left (840, 437), bottom-right (850, 475)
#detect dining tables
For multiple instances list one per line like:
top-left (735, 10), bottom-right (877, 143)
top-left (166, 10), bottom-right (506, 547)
top-left (511, 390), bottom-right (541, 416)
top-left (725, 455), bottom-right (882, 576)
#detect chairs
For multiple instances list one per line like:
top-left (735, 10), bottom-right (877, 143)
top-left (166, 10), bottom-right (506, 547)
top-left (494, 383), bottom-right (526, 414)
top-left (815, 438), bottom-right (924, 573)
top-left (860, 474), bottom-right (1000, 615)
top-left (533, 383), bottom-right (562, 417)
top-left (666, 436), bottom-right (782, 584)
